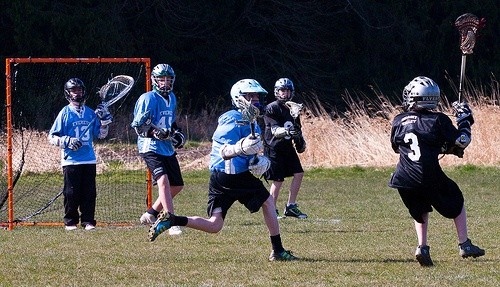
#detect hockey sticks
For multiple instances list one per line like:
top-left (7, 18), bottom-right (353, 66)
top-left (285, 101), bottom-right (303, 126)
top-left (136, 110), bottom-right (178, 144)
top-left (238, 97), bottom-right (260, 165)
top-left (455, 12), bottom-right (479, 103)
top-left (63, 75), bottom-right (135, 161)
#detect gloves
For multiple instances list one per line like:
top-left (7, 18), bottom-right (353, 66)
top-left (60, 136), bottom-right (82, 151)
top-left (248, 155), bottom-right (271, 175)
top-left (452, 99), bottom-right (474, 127)
top-left (235, 133), bottom-right (263, 156)
top-left (151, 128), bottom-right (170, 141)
top-left (294, 137), bottom-right (306, 153)
top-left (285, 123), bottom-right (301, 137)
top-left (171, 130), bottom-right (186, 149)
top-left (94, 101), bottom-right (113, 126)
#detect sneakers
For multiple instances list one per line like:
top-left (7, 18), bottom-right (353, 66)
top-left (268, 248), bottom-right (298, 262)
top-left (458, 238), bottom-right (485, 259)
top-left (64, 226), bottom-right (78, 231)
top-left (284, 203), bottom-right (308, 219)
top-left (415, 245), bottom-right (434, 267)
top-left (85, 224), bottom-right (95, 230)
top-left (274, 209), bottom-right (286, 220)
top-left (147, 211), bottom-right (174, 242)
top-left (168, 225), bottom-right (182, 235)
top-left (139, 211), bottom-right (156, 227)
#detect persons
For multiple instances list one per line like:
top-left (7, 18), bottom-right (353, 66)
top-left (132, 64), bottom-right (186, 236)
top-left (149, 79), bottom-right (300, 262)
top-left (263, 77), bottom-right (307, 219)
top-left (48, 77), bottom-right (111, 230)
top-left (388, 76), bottom-right (485, 268)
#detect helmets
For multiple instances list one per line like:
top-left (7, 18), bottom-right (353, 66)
top-left (151, 63), bottom-right (175, 95)
top-left (64, 77), bottom-right (86, 106)
top-left (401, 76), bottom-right (440, 110)
top-left (230, 77), bottom-right (268, 108)
top-left (274, 78), bottom-right (295, 101)
